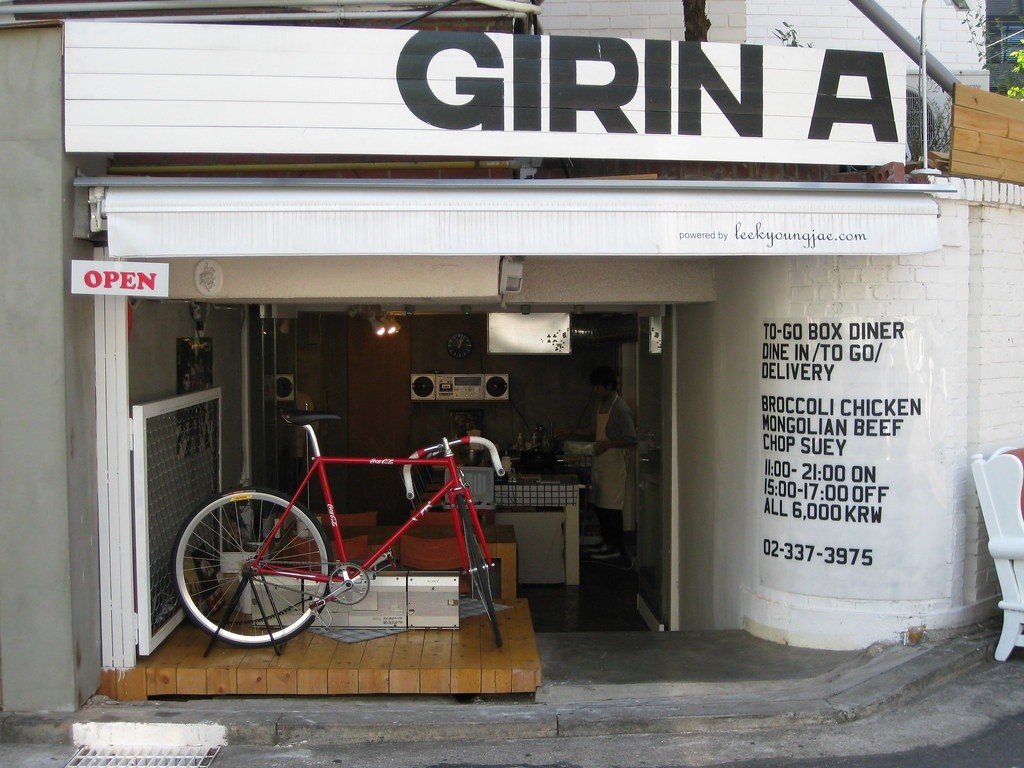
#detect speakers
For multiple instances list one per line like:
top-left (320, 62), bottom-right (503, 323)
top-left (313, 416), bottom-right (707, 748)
top-left (276, 375), bottom-right (295, 400)
top-left (411, 374), bottom-right (435, 400)
top-left (484, 373), bottom-right (509, 400)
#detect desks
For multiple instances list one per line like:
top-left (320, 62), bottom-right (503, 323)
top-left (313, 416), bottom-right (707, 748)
top-left (442, 468), bottom-right (586, 586)
top-left (282, 524), bottom-right (518, 600)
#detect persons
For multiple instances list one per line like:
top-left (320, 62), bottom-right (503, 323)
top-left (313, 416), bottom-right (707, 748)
top-left (555, 364), bottom-right (637, 560)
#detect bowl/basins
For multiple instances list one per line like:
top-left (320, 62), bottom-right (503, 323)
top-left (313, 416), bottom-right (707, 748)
top-left (460, 449), bottom-right (484, 466)
top-left (296, 516), bottom-right (322, 538)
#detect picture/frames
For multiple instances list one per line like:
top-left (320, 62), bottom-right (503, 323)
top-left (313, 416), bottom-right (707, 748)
top-left (448, 409), bottom-right (483, 450)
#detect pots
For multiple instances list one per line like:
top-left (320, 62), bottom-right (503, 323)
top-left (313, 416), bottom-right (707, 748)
top-left (520, 450), bottom-right (565, 471)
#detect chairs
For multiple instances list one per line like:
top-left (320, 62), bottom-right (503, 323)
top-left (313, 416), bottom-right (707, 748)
top-left (400, 534), bottom-right (473, 599)
top-left (413, 455), bottom-right (445, 491)
top-left (311, 511), bottom-right (377, 526)
top-left (411, 509), bottom-right (463, 526)
top-left (971, 445), bottom-right (1024, 663)
top-left (290, 535), bottom-right (369, 570)
top-left (396, 465), bottom-right (443, 512)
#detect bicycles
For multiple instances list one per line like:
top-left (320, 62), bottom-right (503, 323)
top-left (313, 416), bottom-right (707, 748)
top-left (171, 409), bottom-right (504, 661)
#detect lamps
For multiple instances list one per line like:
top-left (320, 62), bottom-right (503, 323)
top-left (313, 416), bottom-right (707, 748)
top-left (378, 311), bottom-right (401, 335)
top-left (367, 309), bottom-right (385, 336)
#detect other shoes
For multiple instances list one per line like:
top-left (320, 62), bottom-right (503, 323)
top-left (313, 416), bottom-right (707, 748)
top-left (584, 542), bottom-right (608, 552)
top-left (590, 544), bottom-right (621, 559)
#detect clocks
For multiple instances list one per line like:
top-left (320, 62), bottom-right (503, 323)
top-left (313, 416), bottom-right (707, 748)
top-left (446, 332), bottom-right (474, 360)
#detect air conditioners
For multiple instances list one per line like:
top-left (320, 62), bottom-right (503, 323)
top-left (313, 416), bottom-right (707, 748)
top-left (840, 70), bottom-right (989, 172)
